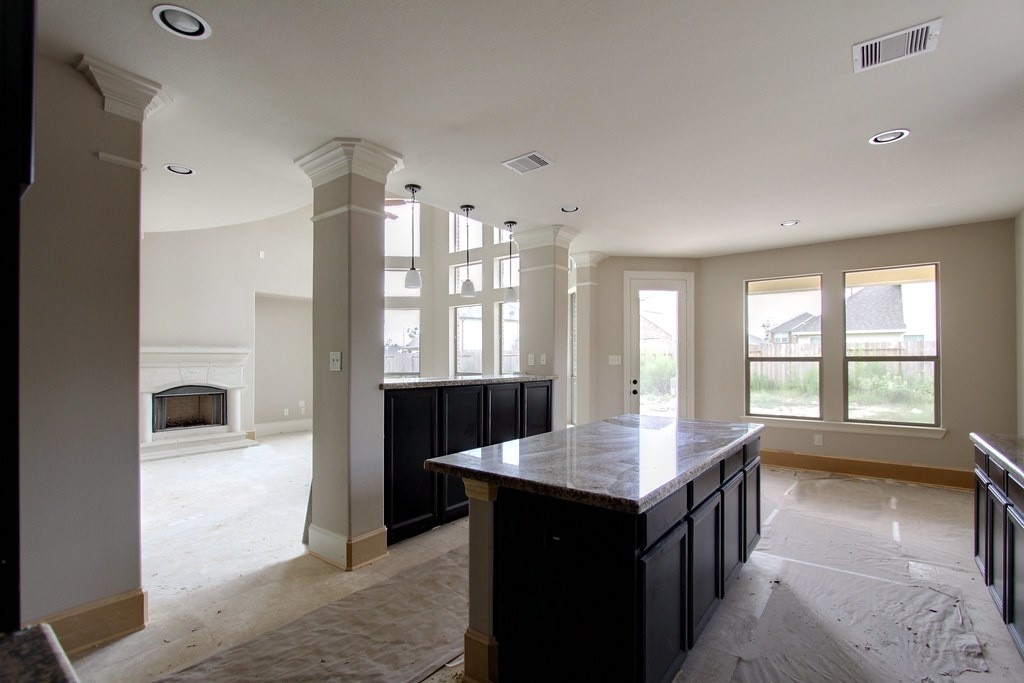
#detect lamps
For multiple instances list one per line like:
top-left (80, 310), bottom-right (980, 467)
top-left (405, 184), bottom-right (423, 288)
top-left (503, 220), bottom-right (517, 302)
top-left (460, 205), bottom-right (476, 297)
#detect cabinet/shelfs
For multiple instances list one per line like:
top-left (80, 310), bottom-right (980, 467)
top-left (968, 433), bottom-right (1024, 660)
top-left (423, 415), bottom-right (765, 683)
top-left (382, 374), bottom-right (554, 545)
